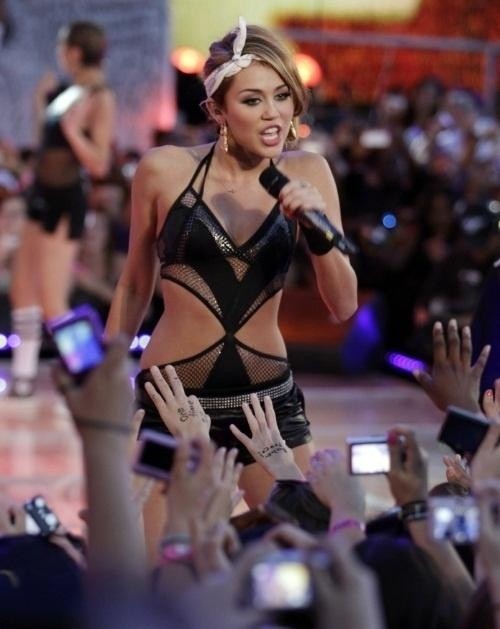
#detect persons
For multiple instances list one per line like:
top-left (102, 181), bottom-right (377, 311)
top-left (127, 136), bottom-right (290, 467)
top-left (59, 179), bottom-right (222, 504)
top-left (101, 24), bottom-right (358, 562)
top-left (0, 73), bottom-right (500, 396)
top-left (0, 320), bottom-right (500, 629)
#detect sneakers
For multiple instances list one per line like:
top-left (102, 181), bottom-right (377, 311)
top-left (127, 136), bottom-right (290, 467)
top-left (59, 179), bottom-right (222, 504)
top-left (2, 395), bottom-right (36, 410)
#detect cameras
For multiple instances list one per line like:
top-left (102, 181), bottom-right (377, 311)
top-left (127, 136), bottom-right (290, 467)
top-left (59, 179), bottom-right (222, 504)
top-left (426, 495), bottom-right (481, 544)
top-left (248, 548), bottom-right (331, 610)
top-left (45, 305), bottom-right (106, 387)
top-left (437, 406), bottom-right (499, 462)
top-left (131, 430), bottom-right (202, 481)
top-left (345, 436), bottom-right (407, 477)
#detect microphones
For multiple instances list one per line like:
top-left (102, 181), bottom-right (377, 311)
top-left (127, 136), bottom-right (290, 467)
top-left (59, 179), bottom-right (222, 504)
top-left (258, 165), bottom-right (350, 256)
